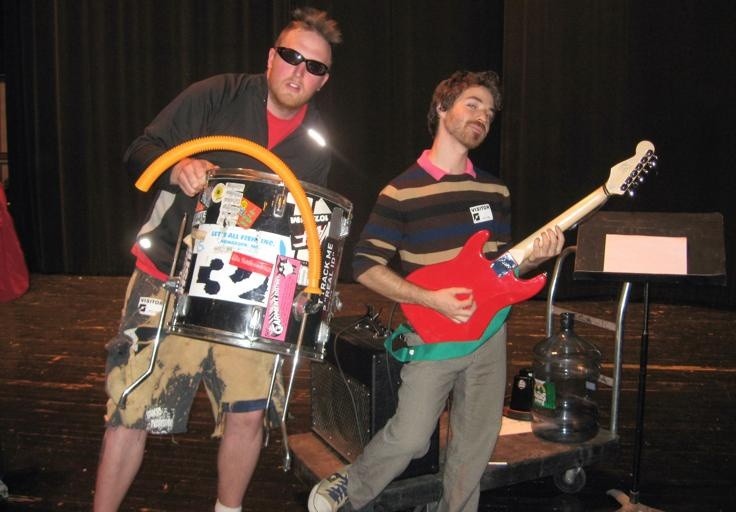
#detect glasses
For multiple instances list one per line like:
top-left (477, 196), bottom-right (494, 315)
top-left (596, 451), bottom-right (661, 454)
top-left (274, 46), bottom-right (329, 77)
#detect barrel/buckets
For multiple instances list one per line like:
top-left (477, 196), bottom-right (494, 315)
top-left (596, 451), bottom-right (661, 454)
top-left (532, 313), bottom-right (602, 444)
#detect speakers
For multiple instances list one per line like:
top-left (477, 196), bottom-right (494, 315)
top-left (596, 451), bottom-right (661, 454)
top-left (311, 314), bottom-right (439, 481)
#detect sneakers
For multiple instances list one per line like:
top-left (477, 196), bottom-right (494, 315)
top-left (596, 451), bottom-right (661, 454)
top-left (307, 463), bottom-right (356, 512)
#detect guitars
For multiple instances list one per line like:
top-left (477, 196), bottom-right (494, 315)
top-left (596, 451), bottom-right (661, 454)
top-left (401, 140), bottom-right (660, 343)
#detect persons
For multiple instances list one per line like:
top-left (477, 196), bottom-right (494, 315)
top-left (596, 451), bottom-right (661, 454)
top-left (91, 6), bottom-right (357, 512)
top-left (305, 67), bottom-right (566, 512)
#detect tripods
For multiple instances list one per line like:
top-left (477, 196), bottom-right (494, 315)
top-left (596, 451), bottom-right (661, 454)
top-left (606, 278), bottom-right (665, 512)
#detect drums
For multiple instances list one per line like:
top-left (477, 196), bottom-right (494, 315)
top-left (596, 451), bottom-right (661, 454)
top-left (166, 167), bottom-right (353, 364)
